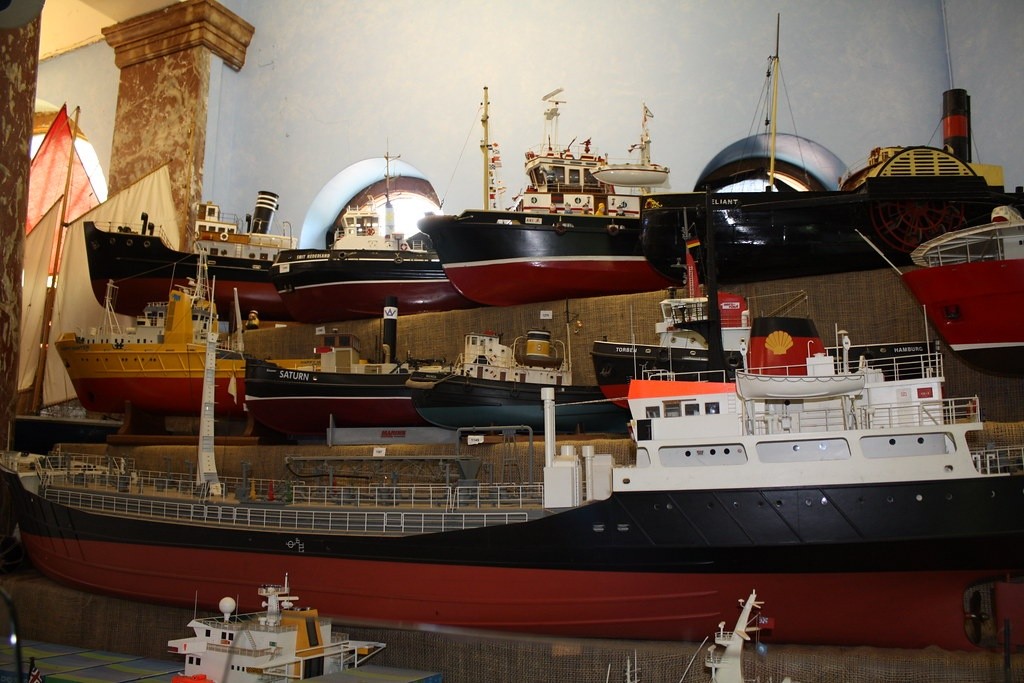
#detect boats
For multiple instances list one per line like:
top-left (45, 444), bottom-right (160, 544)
top-left (264, 135), bottom-right (485, 323)
top-left (50, 246), bottom-right (373, 443)
top-left (27, 570), bottom-right (795, 682)
top-left (6, 302), bottom-right (1024, 652)
top-left (81, 191), bottom-right (288, 324)
top-left (242, 293), bottom-right (462, 444)
top-left (416, 83), bottom-right (681, 306)
top-left (401, 327), bottom-right (630, 433)
top-left (590, 206), bottom-right (826, 409)
top-left (636, 13), bottom-right (1024, 286)
top-left (897, 204), bottom-right (1023, 368)
